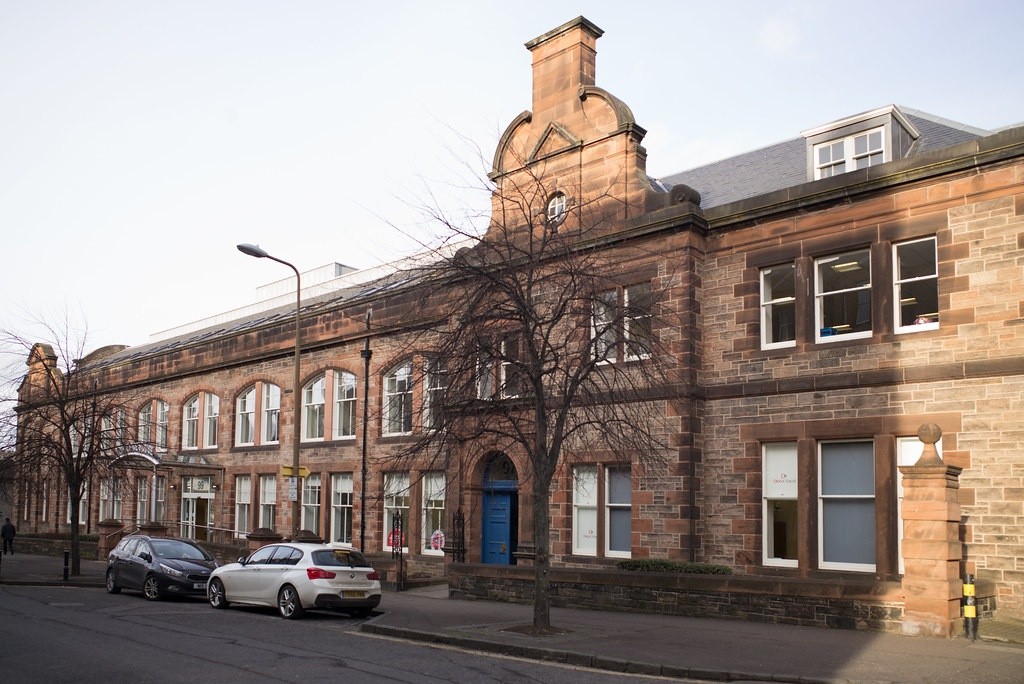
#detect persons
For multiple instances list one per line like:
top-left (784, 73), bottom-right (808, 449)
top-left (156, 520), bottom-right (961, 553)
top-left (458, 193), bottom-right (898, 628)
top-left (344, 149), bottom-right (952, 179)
top-left (1, 518), bottom-right (16, 555)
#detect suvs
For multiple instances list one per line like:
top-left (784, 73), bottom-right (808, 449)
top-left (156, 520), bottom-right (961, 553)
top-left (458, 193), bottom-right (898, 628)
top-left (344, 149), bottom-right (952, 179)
top-left (104, 535), bottom-right (219, 601)
top-left (206, 543), bottom-right (381, 620)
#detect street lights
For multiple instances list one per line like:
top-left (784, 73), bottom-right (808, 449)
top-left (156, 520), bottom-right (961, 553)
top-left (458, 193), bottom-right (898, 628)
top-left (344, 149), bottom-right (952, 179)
top-left (237, 242), bottom-right (304, 543)
